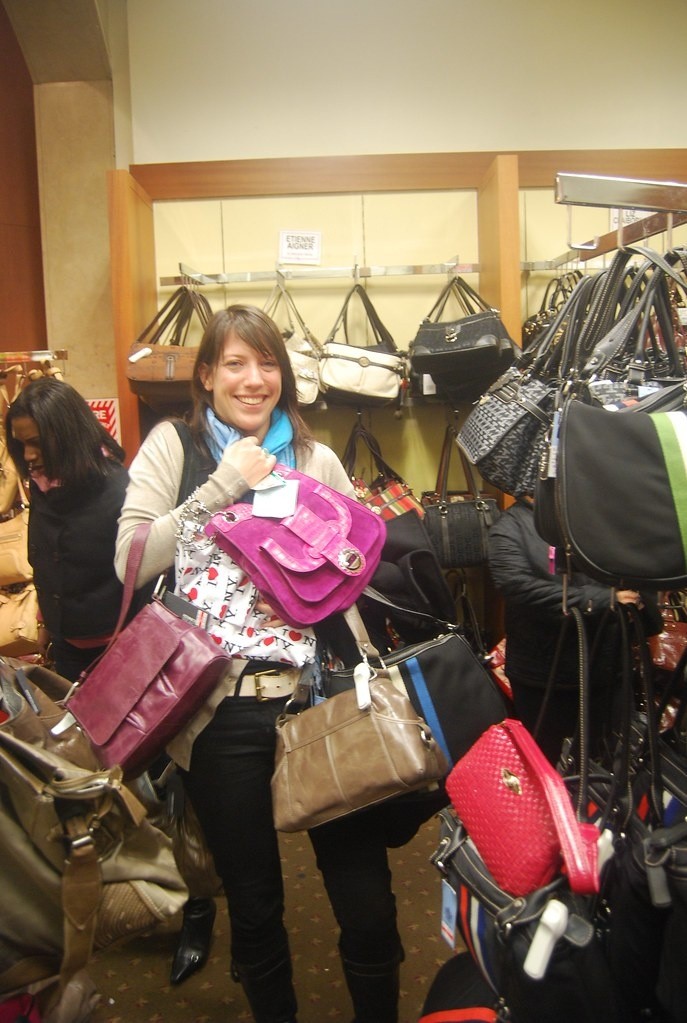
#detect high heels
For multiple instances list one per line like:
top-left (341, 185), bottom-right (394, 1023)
top-left (168, 899), bottom-right (216, 987)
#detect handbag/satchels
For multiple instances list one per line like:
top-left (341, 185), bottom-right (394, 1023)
top-left (315, 582), bottom-right (510, 831)
top-left (0, 657), bottom-right (224, 1020)
top-left (0, 363), bottom-right (70, 658)
top-left (270, 603), bottom-right (450, 836)
top-left (124, 245), bottom-right (686, 666)
top-left (414, 593), bottom-right (687, 1022)
top-left (60, 519), bottom-right (234, 775)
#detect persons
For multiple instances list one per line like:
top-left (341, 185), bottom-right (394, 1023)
top-left (5, 378), bottom-right (217, 989)
top-left (487, 495), bottom-right (643, 773)
top-left (115, 304), bottom-right (406, 1022)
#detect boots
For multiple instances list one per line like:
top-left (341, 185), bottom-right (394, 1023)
top-left (230, 927), bottom-right (299, 1023)
top-left (336, 933), bottom-right (405, 1023)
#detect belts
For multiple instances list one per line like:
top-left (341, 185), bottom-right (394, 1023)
top-left (224, 666), bottom-right (304, 703)
